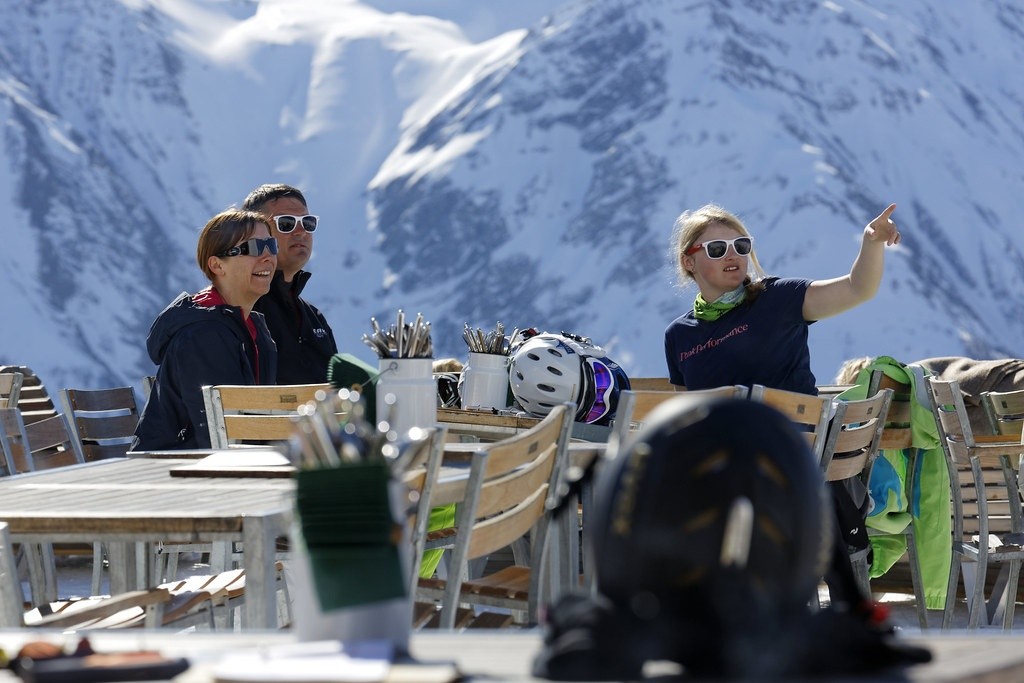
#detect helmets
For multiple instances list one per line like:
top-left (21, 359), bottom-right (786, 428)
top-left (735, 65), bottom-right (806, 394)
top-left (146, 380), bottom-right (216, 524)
top-left (508, 342), bottom-right (631, 428)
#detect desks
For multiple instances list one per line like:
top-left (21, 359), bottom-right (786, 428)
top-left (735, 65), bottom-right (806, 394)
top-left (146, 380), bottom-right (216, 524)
top-left (0, 456), bottom-right (468, 630)
top-left (0, 631), bottom-right (1024, 683)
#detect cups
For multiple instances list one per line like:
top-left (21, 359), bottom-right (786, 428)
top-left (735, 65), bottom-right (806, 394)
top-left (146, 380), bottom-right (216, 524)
top-left (351, 359), bottom-right (438, 444)
top-left (289, 471), bottom-right (415, 650)
top-left (461, 352), bottom-right (510, 412)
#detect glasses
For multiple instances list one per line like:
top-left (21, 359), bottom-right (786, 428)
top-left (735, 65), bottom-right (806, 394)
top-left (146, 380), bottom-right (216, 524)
top-left (584, 357), bottom-right (614, 424)
top-left (270, 214), bottom-right (320, 234)
top-left (224, 237), bottom-right (279, 257)
top-left (685, 237), bottom-right (754, 260)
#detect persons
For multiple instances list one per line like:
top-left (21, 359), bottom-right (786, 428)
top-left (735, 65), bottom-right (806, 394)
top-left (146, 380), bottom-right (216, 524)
top-left (135, 183), bottom-right (338, 454)
top-left (664, 203), bottom-right (902, 637)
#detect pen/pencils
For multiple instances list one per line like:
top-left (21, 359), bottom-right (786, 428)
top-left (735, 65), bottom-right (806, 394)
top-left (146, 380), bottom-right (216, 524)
top-left (462, 321), bottom-right (521, 356)
top-left (361, 308), bottom-right (435, 358)
top-left (270, 385), bottom-right (436, 479)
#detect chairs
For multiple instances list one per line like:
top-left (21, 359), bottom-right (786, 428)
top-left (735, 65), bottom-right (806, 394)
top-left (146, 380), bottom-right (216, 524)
top-left (0, 356), bottom-right (1024, 634)
